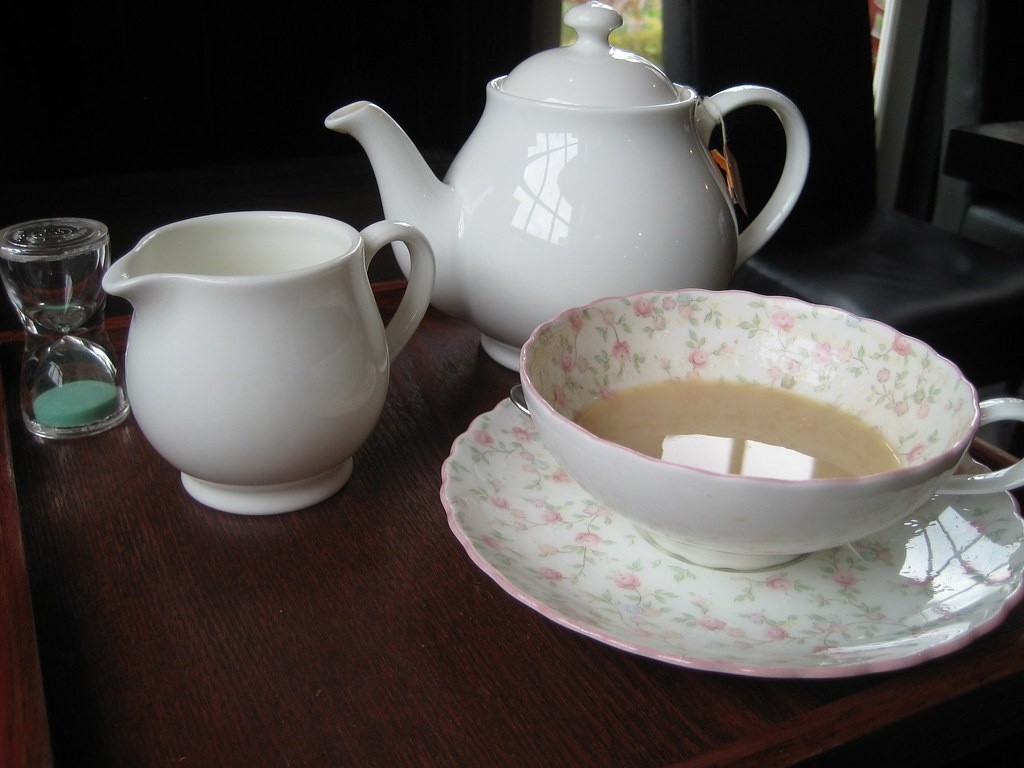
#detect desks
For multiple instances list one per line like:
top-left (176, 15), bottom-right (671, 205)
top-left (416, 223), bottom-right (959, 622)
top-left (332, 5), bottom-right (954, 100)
top-left (0, 268), bottom-right (1024, 768)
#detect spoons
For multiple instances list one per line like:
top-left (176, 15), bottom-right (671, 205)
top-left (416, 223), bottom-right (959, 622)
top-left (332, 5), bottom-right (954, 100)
top-left (509, 383), bottom-right (529, 416)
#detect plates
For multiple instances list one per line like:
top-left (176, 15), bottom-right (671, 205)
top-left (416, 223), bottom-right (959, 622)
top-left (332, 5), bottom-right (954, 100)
top-left (440, 391), bottom-right (1024, 682)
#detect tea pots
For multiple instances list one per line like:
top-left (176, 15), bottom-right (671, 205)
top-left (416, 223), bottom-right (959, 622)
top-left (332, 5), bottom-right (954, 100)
top-left (325, 0), bottom-right (811, 373)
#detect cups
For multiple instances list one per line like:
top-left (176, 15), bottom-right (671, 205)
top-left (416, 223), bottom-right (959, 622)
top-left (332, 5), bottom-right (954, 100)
top-left (101, 210), bottom-right (436, 516)
top-left (520, 288), bottom-right (1024, 571)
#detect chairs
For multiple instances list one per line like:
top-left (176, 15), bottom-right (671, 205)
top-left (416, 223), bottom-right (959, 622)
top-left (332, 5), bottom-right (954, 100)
top-left (661, 1), bottom-right (1024, 380)
